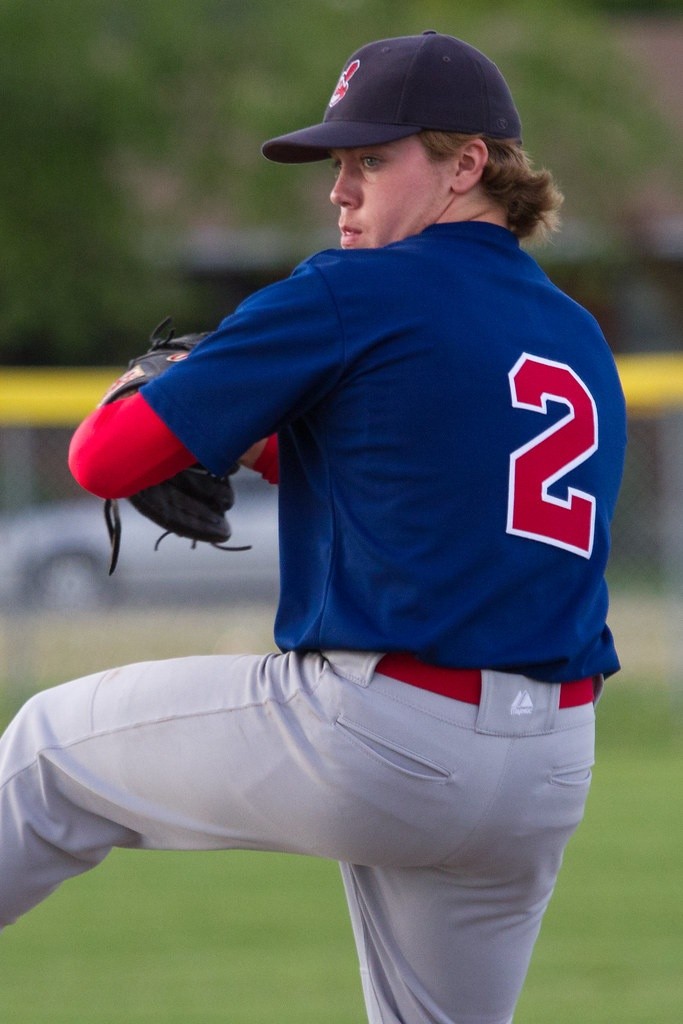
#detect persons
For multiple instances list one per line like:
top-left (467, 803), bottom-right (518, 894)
top-left (2, 32), bottom-right (630, 1024)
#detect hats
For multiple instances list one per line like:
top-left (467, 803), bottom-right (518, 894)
top-left (260, 29), bottom-right (522, 165)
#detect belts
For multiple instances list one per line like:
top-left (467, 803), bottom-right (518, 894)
top-left (374, 647), bottom-right (595, 709)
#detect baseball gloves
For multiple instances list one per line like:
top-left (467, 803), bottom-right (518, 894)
top-left (100, 312), bottom-right (256, 578)
